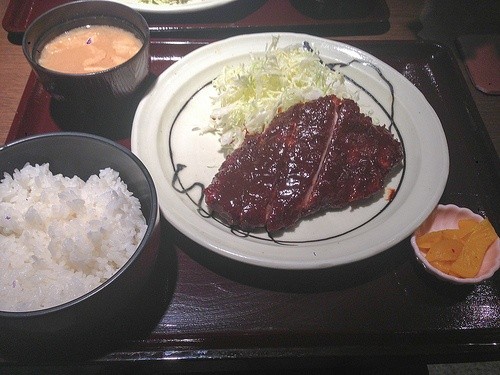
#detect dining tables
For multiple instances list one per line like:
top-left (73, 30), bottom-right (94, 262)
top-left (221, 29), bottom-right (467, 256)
top-left (0, 0), bottom-right (500, 375)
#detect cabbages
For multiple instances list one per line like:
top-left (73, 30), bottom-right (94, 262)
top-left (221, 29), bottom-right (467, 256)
top-left (191, 35), bottom-right (346, 158)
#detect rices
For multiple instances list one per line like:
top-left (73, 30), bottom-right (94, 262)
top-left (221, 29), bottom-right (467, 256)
top-left (0, 161), bottom-right (148, 312)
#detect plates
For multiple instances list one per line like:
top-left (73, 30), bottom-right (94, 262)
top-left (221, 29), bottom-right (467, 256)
top-left (110, 0), bottom-right (235, 14)
top-left (130, 30), bottom-right (450, 270)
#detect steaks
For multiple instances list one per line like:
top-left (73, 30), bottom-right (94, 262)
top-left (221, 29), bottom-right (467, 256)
top-left (202, 94), bottom-right (404, 231)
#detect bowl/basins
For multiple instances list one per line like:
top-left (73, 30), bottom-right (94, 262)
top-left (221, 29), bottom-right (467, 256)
top-left (410, 204), bottom-right (500, 286)
top-left (0, 130), bottom-right (161, 334)
top-left (22, 1), bottom-right (151, 100)
